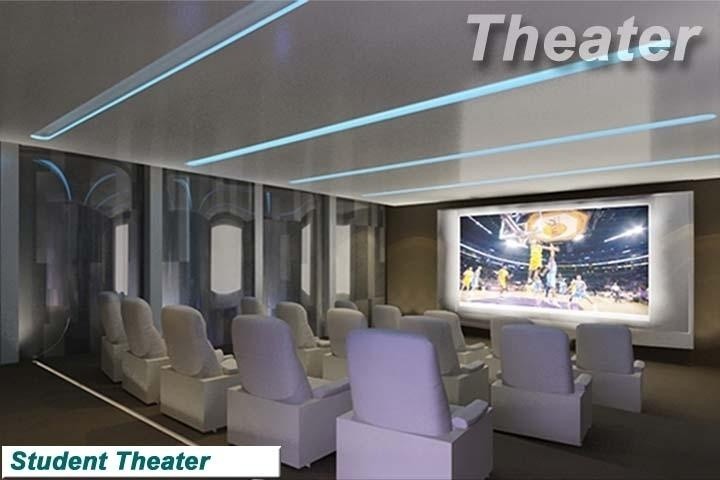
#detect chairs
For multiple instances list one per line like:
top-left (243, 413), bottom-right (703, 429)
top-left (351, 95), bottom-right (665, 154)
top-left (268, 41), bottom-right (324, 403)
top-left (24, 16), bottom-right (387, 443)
top-left (334, 329), bottom-right (494, 480)
top-left (393, 316), bottom-right (490, 405)
top-left (98, 291), bottom-right (128, 383)
top-left (371, 304), bottom-right (403, 329)
top-left (120, 296), bottom-right (171, 404)
top-left (225, 315), bottom-right (352, 469)
top-left (273, 301), bottom-right (331, 379)
top-left (239, 295), bottom-right (268, 316)
top-left (160, 304), bottom-right (242, 433)
top-left (490, 324), bottom-right (594, 447)
top-left (323, 308), bottom-right (368, 382)
top-left (334, 300), bottom-right (359, 312)
top-left (424, 310), bottom-right (501, 384)
top-left (571, 323), bottom-right (646, 414)
top-left (492, 316), bottom-right (535, 357)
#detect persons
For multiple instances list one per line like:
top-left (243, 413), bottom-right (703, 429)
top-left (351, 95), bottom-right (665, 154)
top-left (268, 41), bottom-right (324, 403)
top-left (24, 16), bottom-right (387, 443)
top-left (460, 229), bottom-right (649, 307)
